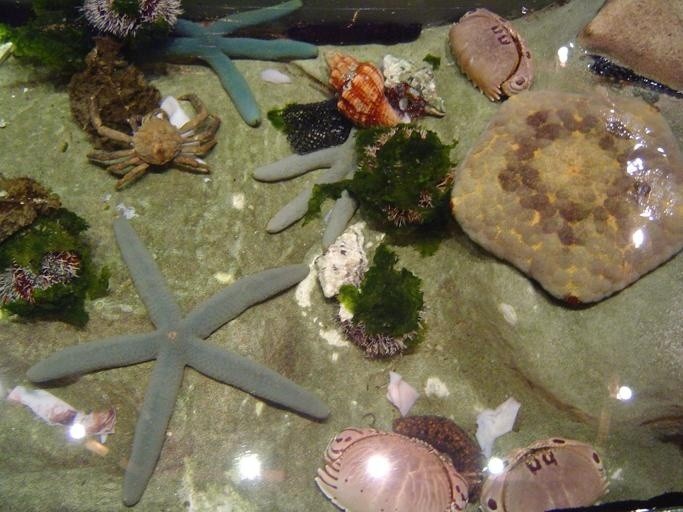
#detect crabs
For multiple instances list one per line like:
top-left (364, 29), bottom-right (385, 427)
top-left (85, 85), bottom-right (222, 193)
